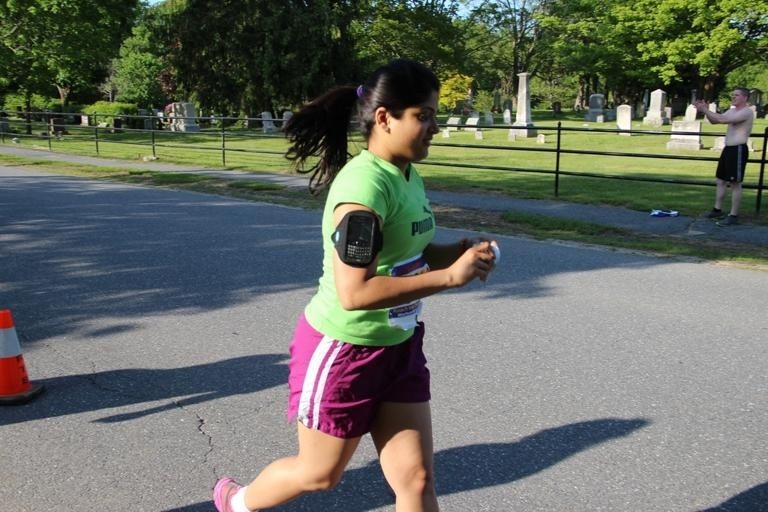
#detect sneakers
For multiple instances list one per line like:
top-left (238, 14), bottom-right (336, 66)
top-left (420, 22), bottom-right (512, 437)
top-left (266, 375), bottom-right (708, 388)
top-left (213, 478), bottom-right (243, 512)
top-left (699, 210), bottom-right (725, 219)
top-left (716, 214), bottom-right (737, 227)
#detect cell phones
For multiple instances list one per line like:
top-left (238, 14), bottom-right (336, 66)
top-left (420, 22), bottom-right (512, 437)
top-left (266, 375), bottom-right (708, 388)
top-left (344, 215), bottom-right (376, 266)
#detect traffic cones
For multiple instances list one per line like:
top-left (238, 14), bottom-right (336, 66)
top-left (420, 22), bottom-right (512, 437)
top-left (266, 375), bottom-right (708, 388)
top-left (0, 307), bottom-right (48, 406)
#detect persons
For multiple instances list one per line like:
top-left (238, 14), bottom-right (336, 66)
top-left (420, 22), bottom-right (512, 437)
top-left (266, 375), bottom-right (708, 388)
top-left (208, 60), bottom-right (499, 511)
top-left (692, 86), bottom-right (755, 226)
